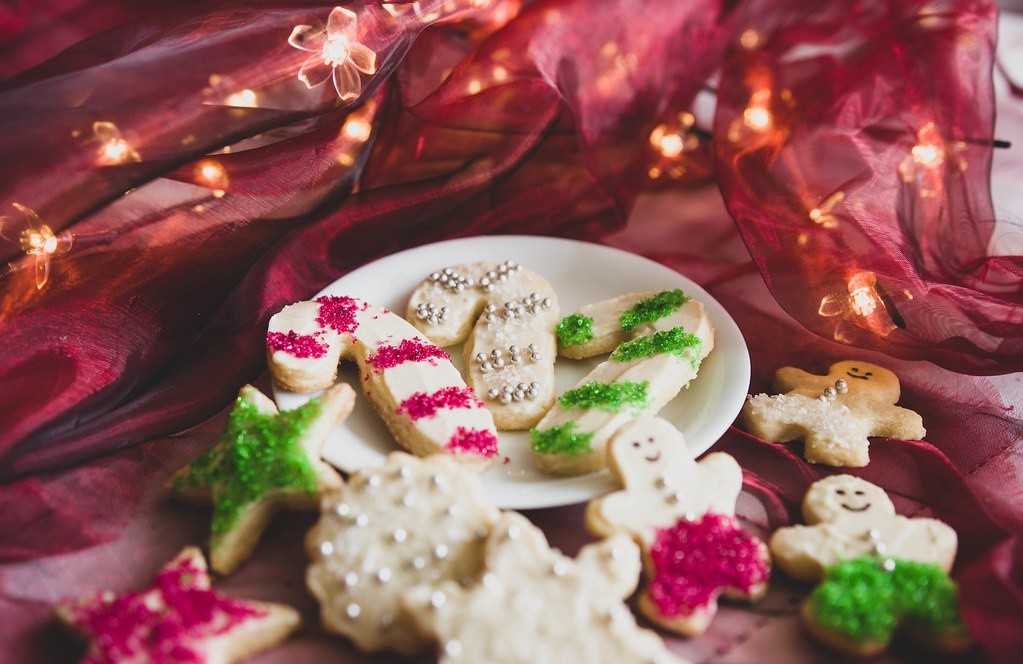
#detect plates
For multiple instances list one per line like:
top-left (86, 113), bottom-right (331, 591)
top-left (271, 232), bottom-right (755, 514)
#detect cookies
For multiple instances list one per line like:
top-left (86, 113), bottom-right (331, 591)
top-left (58, 258), bottom-right (977, 664)
